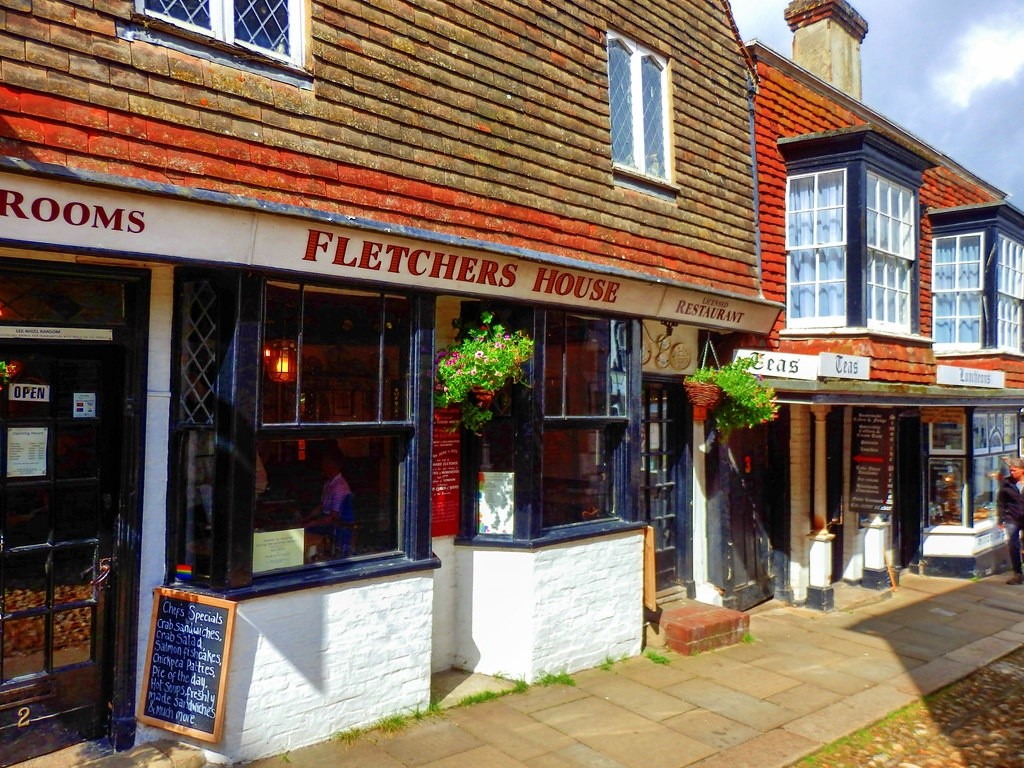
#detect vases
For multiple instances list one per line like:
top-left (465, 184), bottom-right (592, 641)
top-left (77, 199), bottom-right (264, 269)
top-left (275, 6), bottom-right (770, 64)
top-left (684, 379), bottom-right (724, 408)
top-left (470, 386), bottom-right (495, 410)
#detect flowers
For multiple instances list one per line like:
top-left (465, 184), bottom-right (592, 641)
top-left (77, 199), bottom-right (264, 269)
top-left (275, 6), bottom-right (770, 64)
top-left (431, 310), bottom-right (537, 437)
top-left (686, 351), bottom-right (780, 447)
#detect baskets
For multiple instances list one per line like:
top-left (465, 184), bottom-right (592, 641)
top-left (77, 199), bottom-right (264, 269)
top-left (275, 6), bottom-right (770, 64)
top-left (683, 381), bottom-right (722, 410)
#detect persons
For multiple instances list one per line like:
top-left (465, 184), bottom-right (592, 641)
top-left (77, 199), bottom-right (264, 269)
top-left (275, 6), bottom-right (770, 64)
top-left (996, 456), bottom-right (1024, 586)
top-left (298, 438), bottom-right (356, 560)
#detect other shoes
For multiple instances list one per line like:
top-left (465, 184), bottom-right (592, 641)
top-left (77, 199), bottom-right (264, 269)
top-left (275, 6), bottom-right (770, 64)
top-left (1006, 573), bottom-right (1023, 585)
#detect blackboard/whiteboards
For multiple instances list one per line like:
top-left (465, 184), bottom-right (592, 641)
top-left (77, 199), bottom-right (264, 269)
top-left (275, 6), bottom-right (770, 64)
top-left (137, 587), bottom-right (237, 744)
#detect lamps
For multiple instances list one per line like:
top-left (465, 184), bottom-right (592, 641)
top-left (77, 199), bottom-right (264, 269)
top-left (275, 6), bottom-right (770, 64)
top-left (260, 301), bottom-right (301, 382)
